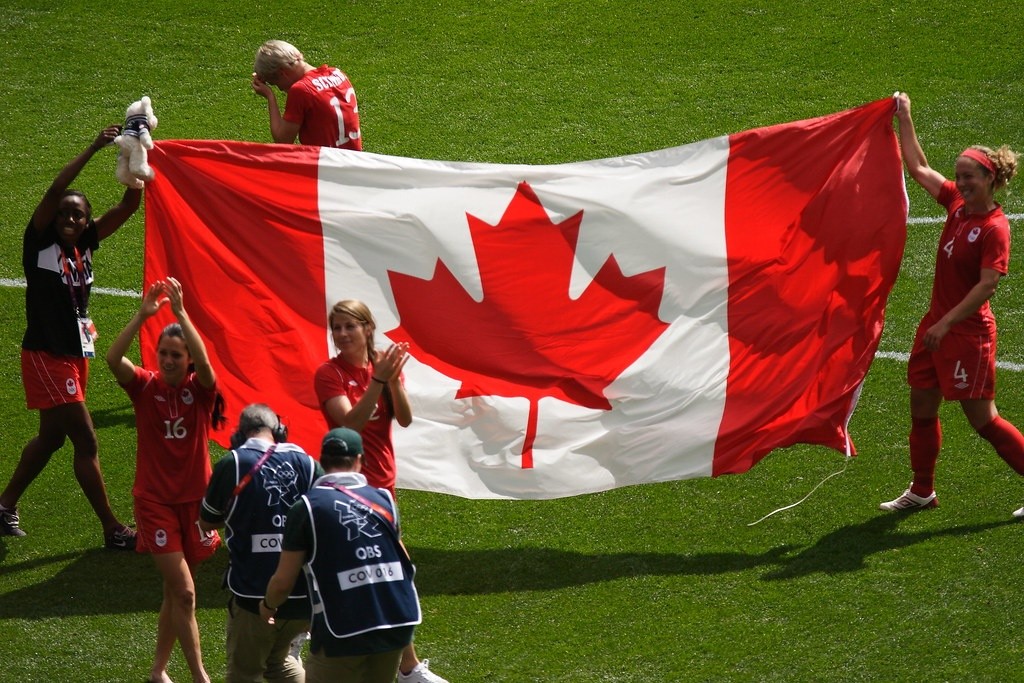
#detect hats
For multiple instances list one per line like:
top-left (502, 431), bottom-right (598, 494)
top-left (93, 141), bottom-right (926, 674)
top-left (322, 428), bottom-right (368, 466)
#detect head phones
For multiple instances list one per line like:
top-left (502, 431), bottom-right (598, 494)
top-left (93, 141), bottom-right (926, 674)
top-left (230, 414), bottom-right (288, 450)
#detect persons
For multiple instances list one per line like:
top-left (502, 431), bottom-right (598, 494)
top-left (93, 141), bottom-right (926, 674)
top-left (105, 276), bottom-right (451, 683)
top-left (879, 90), bottom-right (1024, 525)
top-left (1, 123), bottom-right (143, 551)
top-left (252, 39), bottom-right (362, 151)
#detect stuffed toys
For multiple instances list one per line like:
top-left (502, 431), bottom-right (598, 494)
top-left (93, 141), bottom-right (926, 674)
top-left (114, 96), bottom-right (157, 189)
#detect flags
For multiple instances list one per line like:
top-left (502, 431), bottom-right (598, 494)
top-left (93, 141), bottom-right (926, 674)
top-left (143, 96), bottom-right (913, 502)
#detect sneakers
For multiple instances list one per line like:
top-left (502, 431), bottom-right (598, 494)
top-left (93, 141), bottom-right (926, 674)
top-left (398, 659), bottom-right (449, 683)
top-left (0, 504), bottom-right (26, 536)
top-left (104, 524), bottom-right (138, 551)
top-left (1013, 507), bottom-right (1024, 516)
top-left (880, 482), bottom-right (938, 511)
top-left (288, 632), bottom-right (311, 667)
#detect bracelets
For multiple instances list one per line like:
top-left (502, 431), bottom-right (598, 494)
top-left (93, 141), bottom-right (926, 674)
top-left (371, 376), bottom-right (387, 384)
top-left (262, 597), bottom-right (275, 610)
top-left (90, 143), bottom-right (98, 153)
